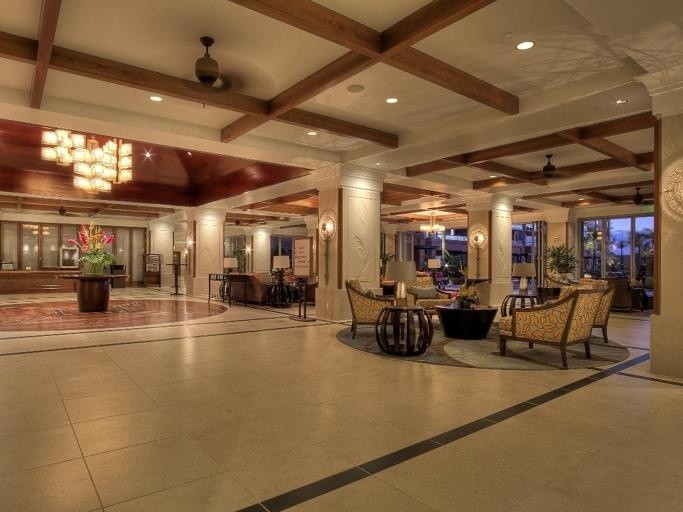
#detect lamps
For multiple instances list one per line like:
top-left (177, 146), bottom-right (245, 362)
top-left (192, 36), bottom-right (220, 91)
top-left (272, 254), bottom-right (290, 286)
top-left (385, 261), bottom-right (416, 307)
top-left (428, 259), bottom-right (442, 281)
top-left (223, 257), bottom-right (238, 273)
top-left (42, 125), bottom-right (133, 195)
top-left (512, 262), bottom-right (537, 291)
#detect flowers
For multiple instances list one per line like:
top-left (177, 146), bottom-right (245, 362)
top-left (453, 282), bottom-right (481, 303)
top-left (67, 220), bottom-right (118, 274)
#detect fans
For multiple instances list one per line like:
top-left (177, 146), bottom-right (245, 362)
top-left (623, 187), bottom-right (655, 206)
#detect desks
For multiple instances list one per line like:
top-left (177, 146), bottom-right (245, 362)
top-left (165, 262), bottom-right (188, 295)
top-left (207, 272), bottom-right (249, 308)
top-left (64, 274), bottom-right (125, 312)
top-left (374, 304), bottom-right (434, 356)
top-left (268, 285), bottom-right (293, 308)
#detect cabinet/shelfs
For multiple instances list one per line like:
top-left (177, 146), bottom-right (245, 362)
top-left (141, 253), bottom-right (162, 288)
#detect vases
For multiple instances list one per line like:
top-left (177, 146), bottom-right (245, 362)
top-left (81, 259), bottom-right (104, 276)
top-left (461, 302), bottom-right (469, 308)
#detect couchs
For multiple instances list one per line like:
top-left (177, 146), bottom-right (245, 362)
top-left (231, 270), bottom-right (273, 303)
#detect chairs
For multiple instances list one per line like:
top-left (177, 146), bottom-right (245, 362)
top-left (296, 275), bottom-right (318, 303)
top-left (343, 277), bottom-right (455, 339)
top-left (497, 274), bottom-right (643, 370)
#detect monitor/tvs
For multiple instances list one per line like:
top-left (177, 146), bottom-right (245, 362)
top-left (60, 247), bottom-right (79, 268)
top-left (1, 263), bottom-right (13, 271)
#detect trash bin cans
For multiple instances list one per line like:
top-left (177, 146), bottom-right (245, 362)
top-left (109, 264), bottom-right (126, 288)
